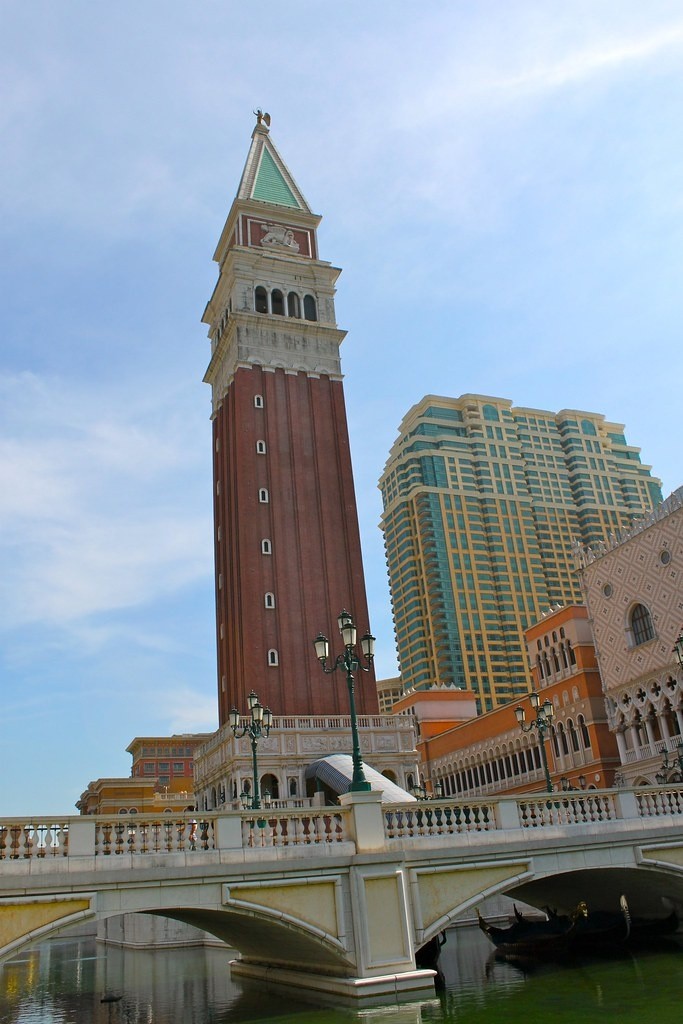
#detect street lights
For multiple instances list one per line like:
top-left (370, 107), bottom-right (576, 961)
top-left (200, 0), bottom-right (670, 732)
top-left (229, 688), bottom-right (274, 828)
top-left (312, 607), bottom-right (380, 791)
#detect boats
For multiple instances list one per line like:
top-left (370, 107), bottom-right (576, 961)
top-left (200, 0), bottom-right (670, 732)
top-left (475, 894), bottom-right (637, 964)
top-left (513, 684), bottom-right (556, 792)
